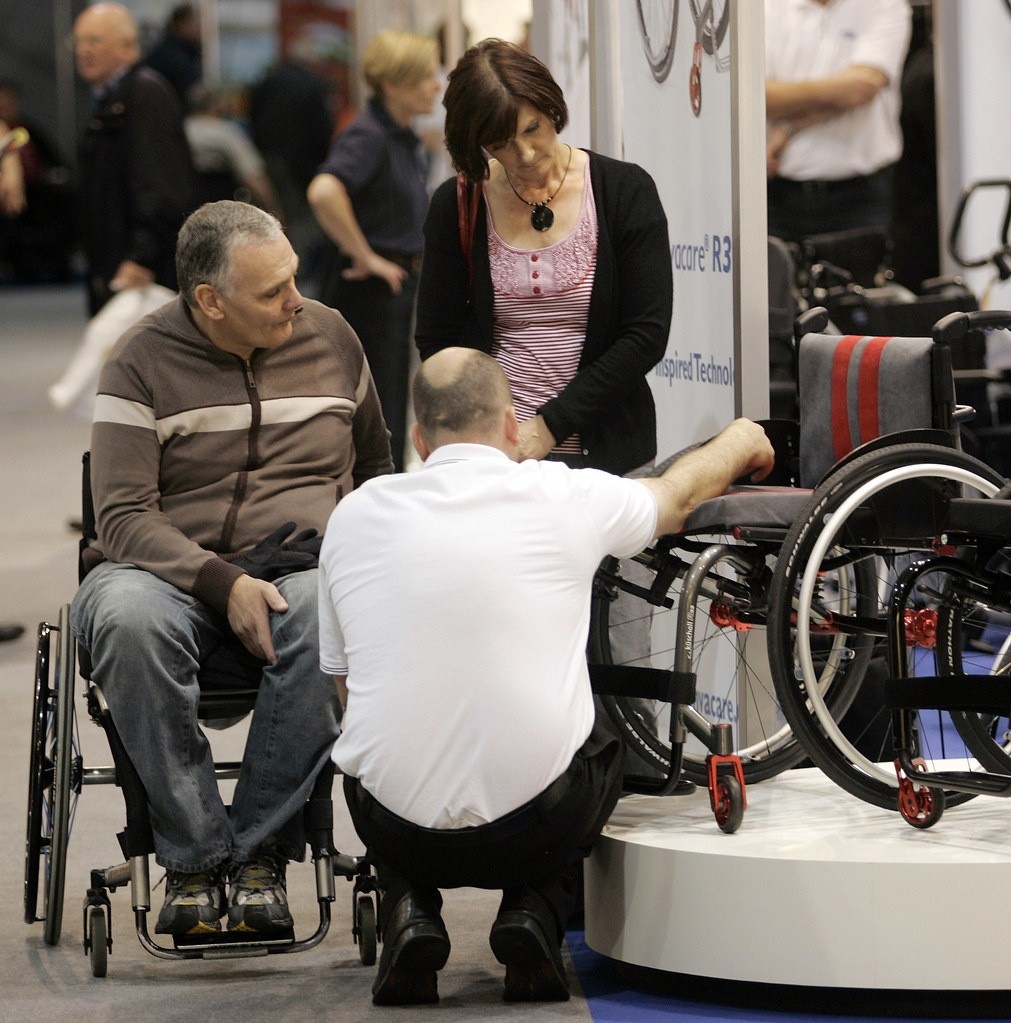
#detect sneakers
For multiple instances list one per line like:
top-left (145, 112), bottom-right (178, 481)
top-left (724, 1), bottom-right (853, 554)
top-left (226, 856), bottom-right (294, 933)
top-left (155, 863), bottom-right (228, 934)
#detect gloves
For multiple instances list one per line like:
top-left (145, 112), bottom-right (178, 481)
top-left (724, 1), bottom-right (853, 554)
top-left (224, 520), bottom-right (325, 580)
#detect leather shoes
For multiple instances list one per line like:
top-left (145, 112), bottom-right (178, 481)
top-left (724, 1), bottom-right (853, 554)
top-left (372, 888), bottom-right (453, 1007)
top-left (488, 885), bottom-right (571, 1005)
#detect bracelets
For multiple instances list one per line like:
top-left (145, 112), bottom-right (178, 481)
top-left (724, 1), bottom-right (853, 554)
top-left (774, 115), bottom-right (792, 136)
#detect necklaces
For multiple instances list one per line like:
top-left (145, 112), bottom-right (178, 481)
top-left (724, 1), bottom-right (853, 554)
top-left (501, 143), bottom-right (584, 233)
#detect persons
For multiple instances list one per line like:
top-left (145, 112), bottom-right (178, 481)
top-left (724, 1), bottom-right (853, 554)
top-left (181, 79), bottom-right (286, 232)
top-left (243, 22), bottom-right (357, 310)
top-left (0, 80), bottom-right (78, 290)
top-left (311, 33), bottom-right (443, 476)
top-left (414, 38), bottom-right (673, 761)
top-left (63, 4), bottom-right (207, 328)
top-left (762, 0), bottom-right (914, 300)
top-left (149, 3), bottom-right (208, 112)
top-left (69, 200), bottom-right (398, 933)
top-left (316, 346), bottom-right (778, 1007)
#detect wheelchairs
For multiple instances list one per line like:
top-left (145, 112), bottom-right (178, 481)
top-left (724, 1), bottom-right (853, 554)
top-left (23, 450), bottom-right (339, 969)
top-left (587, 280), bottom-right (1011, 830)
